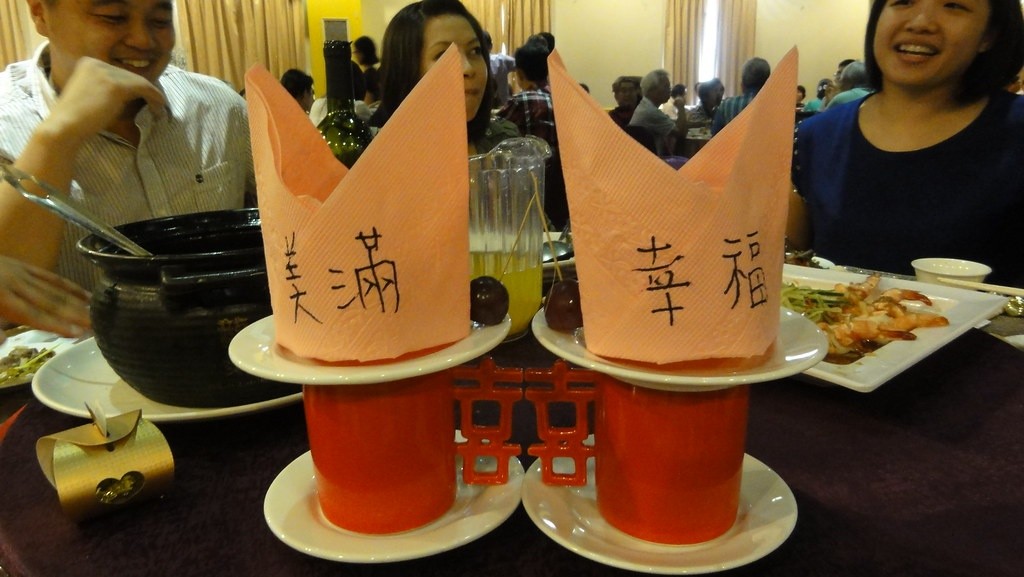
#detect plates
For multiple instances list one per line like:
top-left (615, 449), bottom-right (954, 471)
top-left (263, 449), bottom-right (526, 564)
top-left (0, 322), bottom-right (73, 391)
top-left (532, 304), bottom-right (829, 393)
top-left (782, 259), bottom-right (1010, 393)
top-left (471, 232), bottom-right (581, 270)
top-left (31, 334), bottom-right (303, 426)
top-left (522, 432), bottom-right (799, 574)
top-left (785, 252), bottom-right (836, 269)
top-left (228, 310), bottom-right (511, 387)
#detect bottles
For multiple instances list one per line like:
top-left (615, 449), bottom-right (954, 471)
top-left (311, 40), bottom-right (375, 169)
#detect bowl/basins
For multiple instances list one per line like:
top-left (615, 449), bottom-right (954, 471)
top-left (910, 258), bottom-right (992, 285)
top-left (75, 209), bottom-right (313, 406)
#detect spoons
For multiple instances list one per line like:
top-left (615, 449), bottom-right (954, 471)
top-left (539, 220), bottom-right (574, 262)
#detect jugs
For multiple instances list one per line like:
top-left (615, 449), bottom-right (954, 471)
top-left (467, 133), bottom-right (550, 340)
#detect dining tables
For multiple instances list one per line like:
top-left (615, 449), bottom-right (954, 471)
top-left (0, 284), bottom-right (1024, 577)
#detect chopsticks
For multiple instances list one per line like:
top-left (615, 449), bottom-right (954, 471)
top-left (937, 277), bottom-right (1024, 296)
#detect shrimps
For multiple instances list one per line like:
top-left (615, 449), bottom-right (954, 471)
top-left (821, 272), bottom-right (950, 354)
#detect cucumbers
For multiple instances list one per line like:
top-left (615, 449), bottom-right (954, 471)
top-left (778, 282), bottom-right (847, 323)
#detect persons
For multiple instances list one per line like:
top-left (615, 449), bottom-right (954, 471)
top-left (785, 0), bottom-right (1024, 288)
top-left (0, 255), bottom-right (92, 346)
top-left (241, 0), bottom-right (1024, 233)
top-left (0, 0), bottom-right (258, 322)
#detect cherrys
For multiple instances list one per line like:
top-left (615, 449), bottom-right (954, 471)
top-left (470, 170), bottom-right (585, 331)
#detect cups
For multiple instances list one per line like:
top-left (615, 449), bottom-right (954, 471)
top-left (300, 355), bottom-right (526, 533)
top-left (525, 356), bottom-right (752, 545)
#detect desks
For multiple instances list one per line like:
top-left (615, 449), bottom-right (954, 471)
top-left (680, 128), bottom-right (712, 159)
